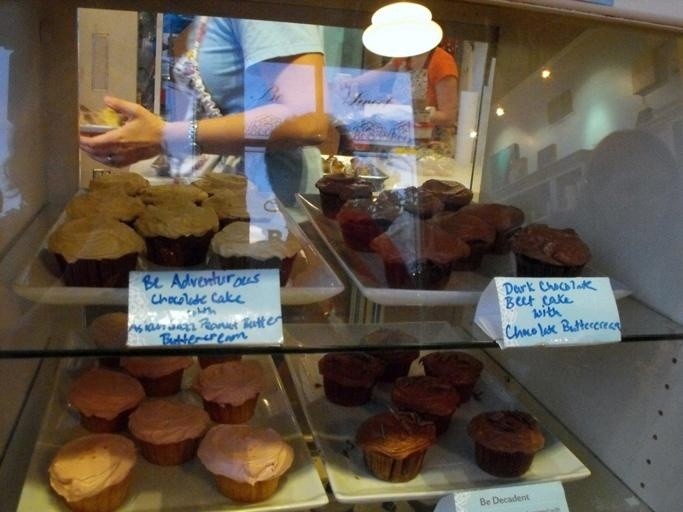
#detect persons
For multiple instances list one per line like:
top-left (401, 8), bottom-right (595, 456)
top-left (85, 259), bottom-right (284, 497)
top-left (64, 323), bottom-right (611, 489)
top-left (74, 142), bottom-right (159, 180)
top-left (343, 44), bottom-right (462, 152)
top-left (80, 8), bottom-right (329, 209)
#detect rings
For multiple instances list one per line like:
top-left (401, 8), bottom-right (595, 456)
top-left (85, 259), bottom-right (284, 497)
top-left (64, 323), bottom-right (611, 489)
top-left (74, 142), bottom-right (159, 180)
top-left (106, 153), bottom-right (115, 167)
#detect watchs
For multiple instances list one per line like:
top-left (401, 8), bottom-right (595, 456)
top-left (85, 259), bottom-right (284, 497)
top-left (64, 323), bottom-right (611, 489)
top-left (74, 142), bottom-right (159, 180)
top-left (184, 120), bottom-right (205, 159)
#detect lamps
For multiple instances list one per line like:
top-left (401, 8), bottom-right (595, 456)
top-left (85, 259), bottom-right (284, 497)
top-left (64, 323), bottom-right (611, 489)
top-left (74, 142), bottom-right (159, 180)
top-left (362, 2), bottom-right (445, 59)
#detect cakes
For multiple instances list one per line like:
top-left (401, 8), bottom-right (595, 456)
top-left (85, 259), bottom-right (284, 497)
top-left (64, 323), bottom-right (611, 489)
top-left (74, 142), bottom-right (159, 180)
top-left (314, 172), bottom-right (593, 293)
top-left (316, 327), bottom-right (546, 484)
top-left (41, 164), bottom-right (303, 289)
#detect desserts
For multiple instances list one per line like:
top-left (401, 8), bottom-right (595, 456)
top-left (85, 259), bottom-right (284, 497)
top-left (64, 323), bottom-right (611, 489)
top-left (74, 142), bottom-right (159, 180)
top-left (46, 313), bottom-right (297, 512)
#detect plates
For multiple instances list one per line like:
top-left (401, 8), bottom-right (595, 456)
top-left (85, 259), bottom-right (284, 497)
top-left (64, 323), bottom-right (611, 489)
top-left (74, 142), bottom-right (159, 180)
top-left (15, 329), bottom-right (328, 512)
top-left (15, 186), bottom-right (345, 309)
top-left (79, 124), bottom-right (121, 137)
top-left (293, 191), bottom-right (636, 309)
top-left (283, 347), bottom-right (594, 505)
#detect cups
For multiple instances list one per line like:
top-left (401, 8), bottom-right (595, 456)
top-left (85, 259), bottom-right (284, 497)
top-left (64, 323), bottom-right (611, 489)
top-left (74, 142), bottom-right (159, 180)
top-left (57, 341), bottom-right (282, 512)
top-left (44, 191), bottom-right (293, 287)
top-left (318, 189), bottom-right (588, 288)
top-left (321, 354), bottom-right (538, 484)
